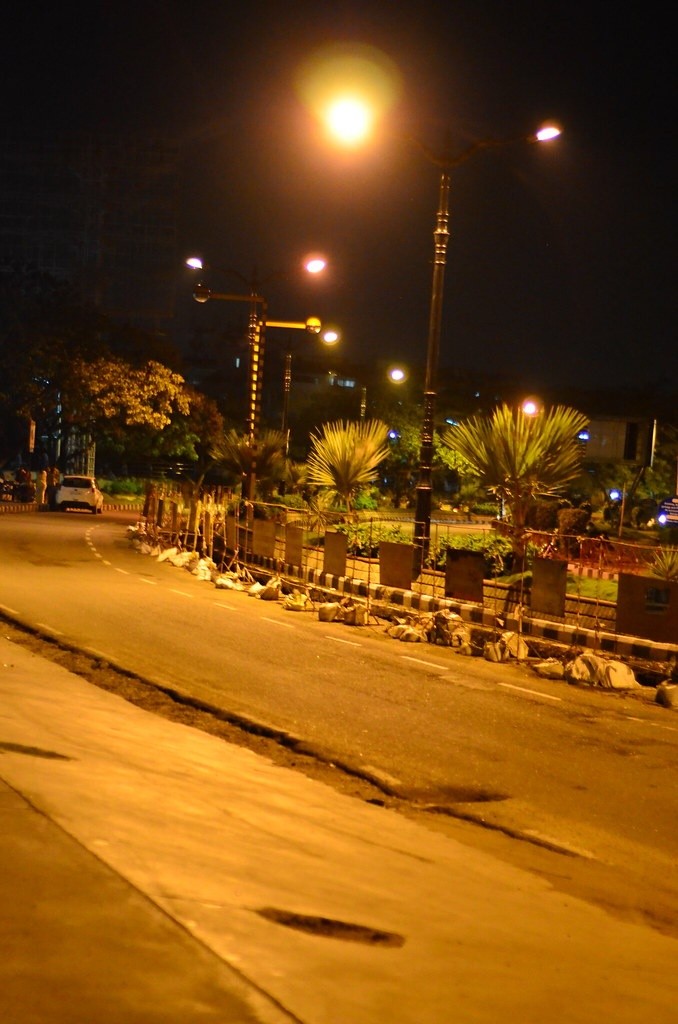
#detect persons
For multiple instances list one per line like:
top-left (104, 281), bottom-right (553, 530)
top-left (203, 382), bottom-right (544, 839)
top-left (37, 463), bottom-right (59, 510)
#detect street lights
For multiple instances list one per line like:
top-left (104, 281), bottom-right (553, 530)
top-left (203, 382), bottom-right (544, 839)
top-left (186, 255), bottom-right (324, 437)
top-left (193, 280), bottom-right (268, 447)
top-left (250, 316), bottom-right (321, 502)
top-left (322, 91), bottom-right (566, 568)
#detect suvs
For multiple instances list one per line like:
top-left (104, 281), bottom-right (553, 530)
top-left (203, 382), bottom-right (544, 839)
top-left (54, 475), bottom-right (104, 515)
